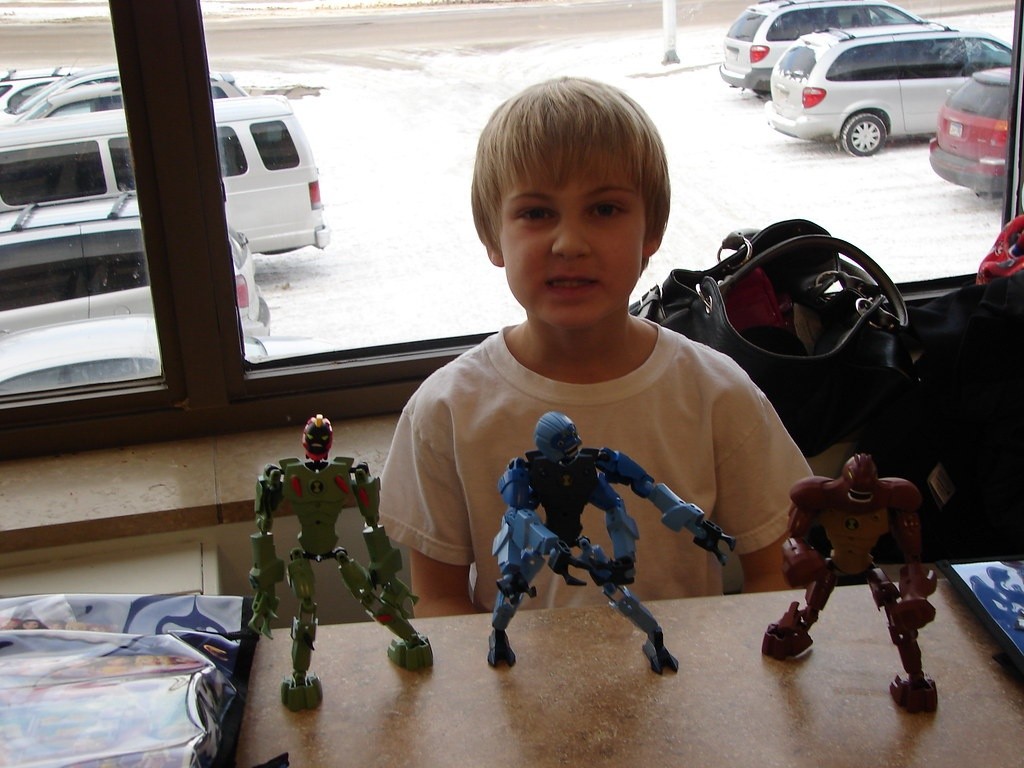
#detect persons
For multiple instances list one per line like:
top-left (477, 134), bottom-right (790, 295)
top-left (763, 450), bottom-right (947, 717)
top-left (245, 414), bottom-right (435, 717)
top-left (481, 411), bottom-right (737, 676)
top-left (367, 74), bottom-right (819, 614)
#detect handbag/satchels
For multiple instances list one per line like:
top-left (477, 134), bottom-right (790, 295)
top-left (624, 218), bottom-right (919, 463)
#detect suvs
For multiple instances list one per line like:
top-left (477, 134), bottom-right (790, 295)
top-left (1, 190), bottom-right (274, 389)
top-left (718, 0), bottom-right (931, 95)
top-left (4, 66), bottom-right (247, 121)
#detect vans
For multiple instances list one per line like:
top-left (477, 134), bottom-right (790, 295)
top-left (0, 95), bottom-right (329, 256)
top-left (763, 25), bottom-right (1013, 157)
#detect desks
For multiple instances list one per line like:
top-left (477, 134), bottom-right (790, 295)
top-left (237, 562), bottom-right (1023, 768)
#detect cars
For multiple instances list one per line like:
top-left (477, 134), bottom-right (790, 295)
top-left (929, 64), bottom-right (1010, 201)
top-left (0, 69), bottom-right (82, 118)
